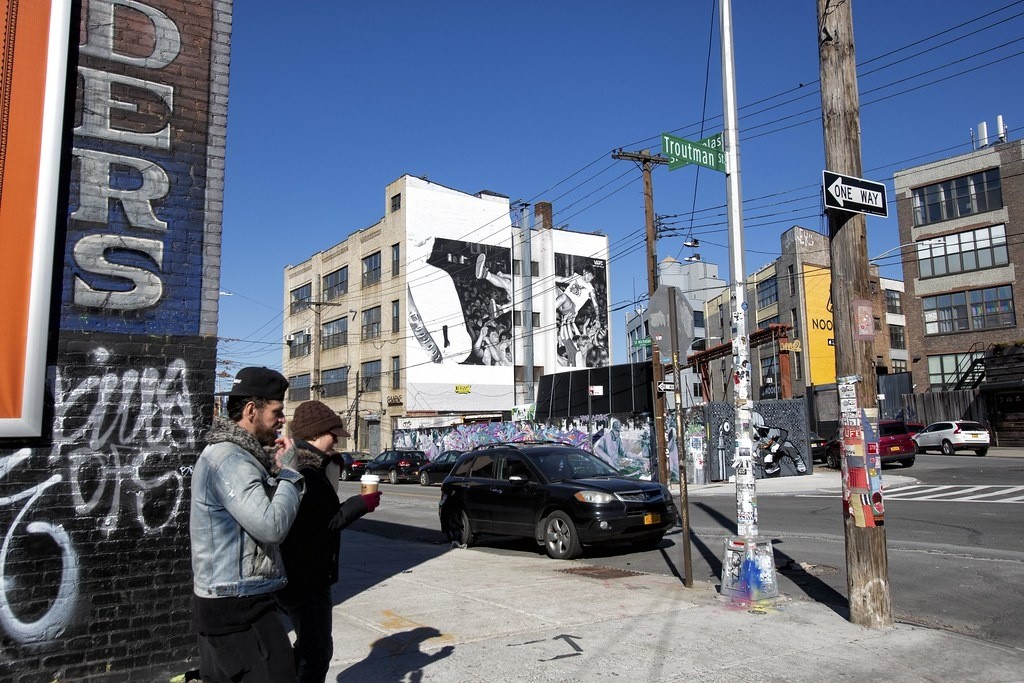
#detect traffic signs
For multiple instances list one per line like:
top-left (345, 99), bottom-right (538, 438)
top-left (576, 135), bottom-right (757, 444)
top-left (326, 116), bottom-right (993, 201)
top-left (822, 170), bottom-right (889, 218)
top-left (657, 380), bottom-right (676, 394)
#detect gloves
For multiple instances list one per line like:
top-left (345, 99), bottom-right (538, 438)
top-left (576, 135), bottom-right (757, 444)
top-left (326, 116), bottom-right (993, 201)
top-left (359, 491), bottom-right (382, 512)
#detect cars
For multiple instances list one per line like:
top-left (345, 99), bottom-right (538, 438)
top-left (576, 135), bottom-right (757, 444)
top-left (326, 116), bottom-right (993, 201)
top-left (905, 423), bottom-right (926, 446)
top-left (810, 431), bottom-right (828, 463)
top-left (911, 419), bottom-right (992, 457)
top-left (338, 451), bottom-right (375, 482)
top-left (418, 450), bottom-right (471, 486)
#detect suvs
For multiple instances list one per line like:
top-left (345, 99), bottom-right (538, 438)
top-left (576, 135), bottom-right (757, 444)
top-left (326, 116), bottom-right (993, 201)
top-left (822, 418), bottom-right (916, 469)
top-left (362, 448), bottom-right (432, 485)
top-left (436, 438), bottom-right (680, 561)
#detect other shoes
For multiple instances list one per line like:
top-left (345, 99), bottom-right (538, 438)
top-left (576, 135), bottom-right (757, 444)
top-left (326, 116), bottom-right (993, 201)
top-left (476, 253), bottom-right (487, 280)
top-left (489, 299), bottom-right (501, 319)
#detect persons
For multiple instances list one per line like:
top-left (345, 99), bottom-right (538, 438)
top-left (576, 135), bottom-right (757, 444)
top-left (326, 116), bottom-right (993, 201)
top-left (453, 253), bottom-right (610, 367)
top-left (189, 366), bottom-right (306, 683)
top-left (263, 400), bottom-right (382, 683)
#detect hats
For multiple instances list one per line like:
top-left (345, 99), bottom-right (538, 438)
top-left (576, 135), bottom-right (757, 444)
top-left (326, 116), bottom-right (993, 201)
top-left (212, 366), bottom-right (290, 401)
top-left (290, 400), bottom-right (351, 441)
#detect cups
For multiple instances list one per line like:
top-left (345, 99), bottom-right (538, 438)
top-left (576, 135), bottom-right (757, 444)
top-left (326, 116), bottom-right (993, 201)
top-left (360, 475), bottom-right (381, 494)
